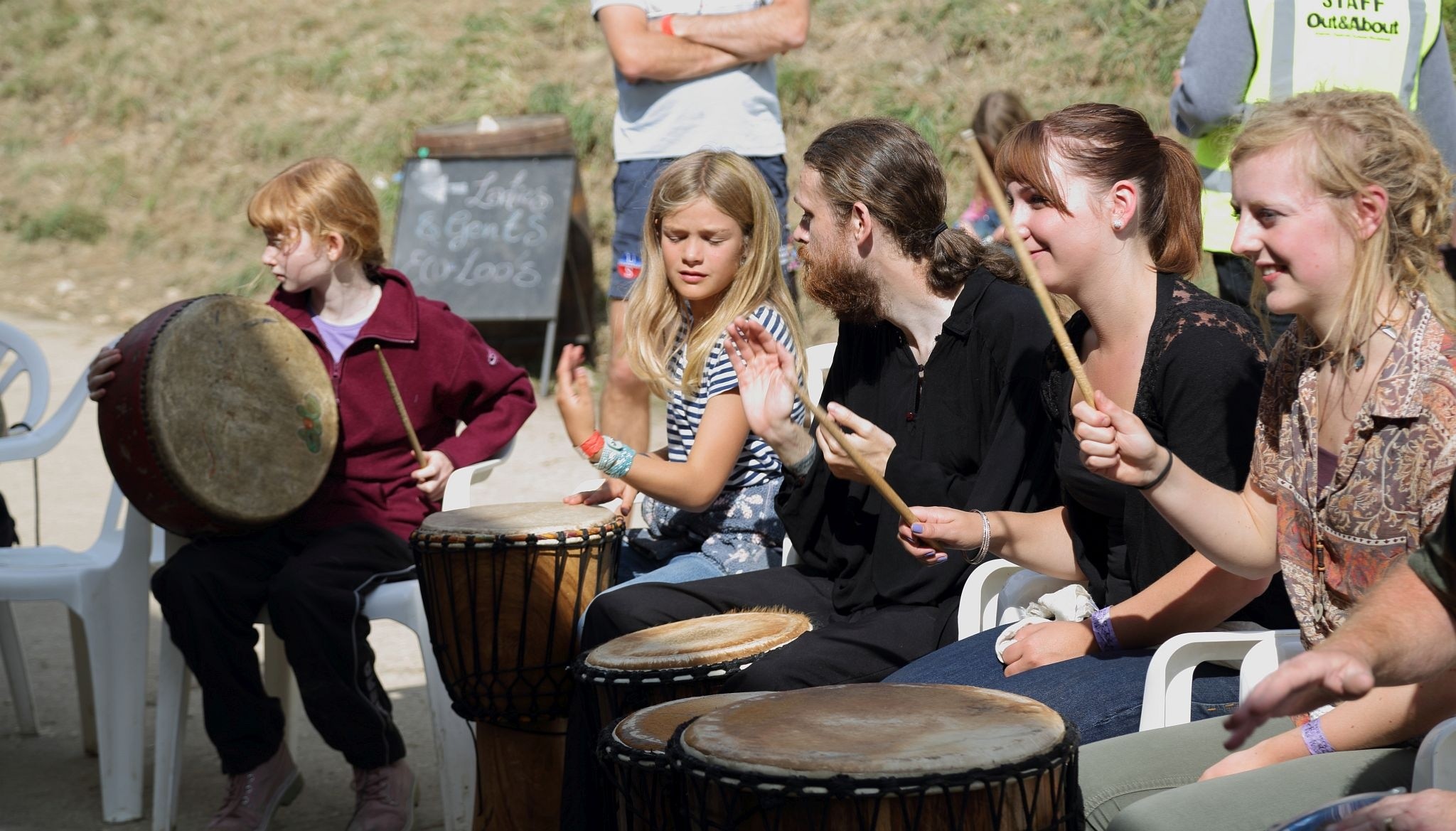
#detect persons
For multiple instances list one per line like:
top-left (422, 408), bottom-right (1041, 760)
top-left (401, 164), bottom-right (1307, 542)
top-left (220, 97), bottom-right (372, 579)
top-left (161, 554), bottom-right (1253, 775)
top-left (555, 91), bottom-right (1456, 831)
top-left (85, 156), bottom-right (535, 831)
top-left (1170, 0), bottom-right (1456, 358)
top-left (591, 0), bottom-right (809, 477)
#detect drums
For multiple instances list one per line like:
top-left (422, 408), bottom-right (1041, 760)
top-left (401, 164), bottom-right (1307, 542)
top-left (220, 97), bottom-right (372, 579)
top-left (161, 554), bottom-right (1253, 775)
top-left (97, 293), bottom-right (342, 546)
top-left (564, 604), bottom-right (814, 736)
top-left (596, 690), bottom-right (782, 831)
top-left (659, 682), bottom-right (1070, 831)
top-left (409, 501), bottom-right (626, 726)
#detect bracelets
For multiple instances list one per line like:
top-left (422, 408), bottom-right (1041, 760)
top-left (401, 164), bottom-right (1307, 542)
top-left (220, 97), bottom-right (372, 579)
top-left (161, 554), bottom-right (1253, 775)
top-left (580, 436), bottom-right (604, 459)
top-left (573, 432), bottom-right (599, 454)
top-left (661, 14), bottom-right (672, 35)
top-left (1300, 717), bottom-right (1338, 755)
top-left (1091, 605), bottom-right (1120, 653)
top-left (968, 512), bottom-right (991, 565)
top-left (962, 509), bottom-right (986, 561)
top-left (1132, 446), bottom-right (1173, 489)
top-left (963, 511), bottom-right (988, 564)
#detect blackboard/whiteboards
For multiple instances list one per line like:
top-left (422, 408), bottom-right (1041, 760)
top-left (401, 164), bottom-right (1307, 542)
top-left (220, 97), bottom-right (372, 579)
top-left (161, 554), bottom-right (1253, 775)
top-left (389, 154), bottom-right (580, 325)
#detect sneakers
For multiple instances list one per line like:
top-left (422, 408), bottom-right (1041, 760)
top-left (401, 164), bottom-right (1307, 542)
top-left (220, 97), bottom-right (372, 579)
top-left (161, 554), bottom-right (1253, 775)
top-left (346, 758), bottom-right (420, 831)
top-left (204, 738), bottom-right (304, 831)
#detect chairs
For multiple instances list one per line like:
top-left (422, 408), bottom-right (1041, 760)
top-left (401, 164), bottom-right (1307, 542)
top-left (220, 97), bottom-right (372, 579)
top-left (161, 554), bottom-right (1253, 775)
top-left (0, 318), bottom-right (1314, 830)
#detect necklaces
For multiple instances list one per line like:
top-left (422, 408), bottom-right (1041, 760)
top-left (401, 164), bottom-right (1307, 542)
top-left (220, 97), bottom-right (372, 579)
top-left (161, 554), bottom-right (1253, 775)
top-left (1313, 289), bottom-right (1400, 373)
